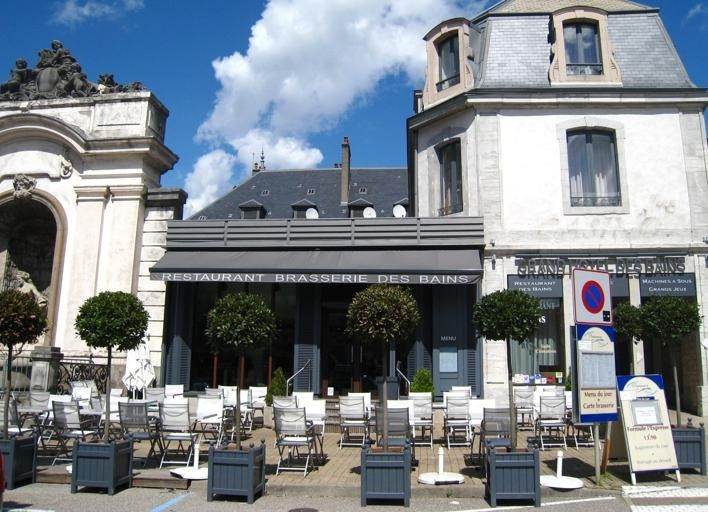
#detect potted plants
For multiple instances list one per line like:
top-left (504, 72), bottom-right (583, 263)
top-left (0, 289), bottom-right (47, 489)
top-left (69, 290), bottom-right (148, 493)
top-left (612, 292), bottom-right (706, 478)
top-left (345, 282), bottom-right (420, 508)
top-left (203, 293), bottom-right (280, 504)
top-left (469, 289), bottom-right (541, 507)
